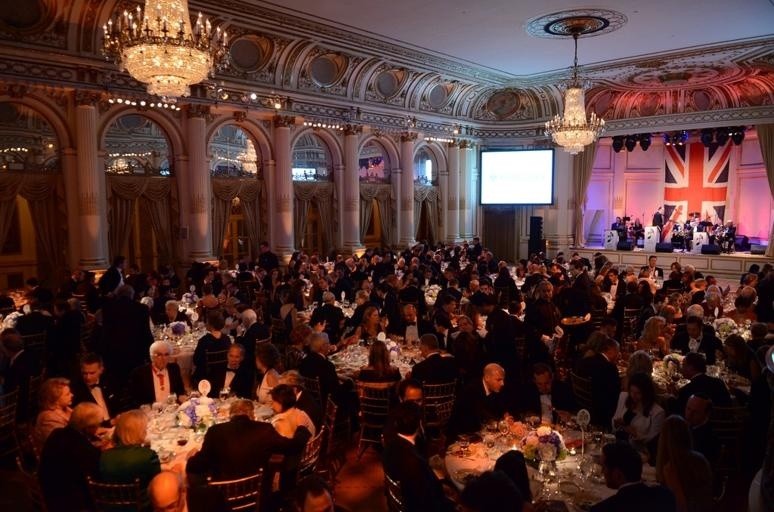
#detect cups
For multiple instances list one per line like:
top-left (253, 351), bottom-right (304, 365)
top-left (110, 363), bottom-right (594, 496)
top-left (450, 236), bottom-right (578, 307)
top-left (345, 299), bottom-right (357, 311)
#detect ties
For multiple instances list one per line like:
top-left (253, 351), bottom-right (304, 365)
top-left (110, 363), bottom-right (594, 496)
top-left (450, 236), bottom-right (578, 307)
top-left (157, 373), bottom-right (165, 392)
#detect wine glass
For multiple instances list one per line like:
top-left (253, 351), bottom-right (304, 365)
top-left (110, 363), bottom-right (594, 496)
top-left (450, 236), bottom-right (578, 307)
top-left (458, 418), bottom-right (619, 511)
top-left (671, 372), bottom-right (680, 387)
top-left (142, 388), bottom-right (230, 466)
top-left (192, 333), bottom-right (198, 347)
top-left (702, 315), bottom-right (752, 343)
top-left (331, 337), bottom-right (421, 374)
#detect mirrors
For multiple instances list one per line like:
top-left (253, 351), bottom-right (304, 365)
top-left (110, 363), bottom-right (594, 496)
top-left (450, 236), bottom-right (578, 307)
top-left (1, 99), bottom-right (61, 174)
top-left (291, 131), bottom-right (335, 183)
top-left (414, 144), bottom-right (440, 187)
top-left (359, 138), bottom-right (393, 185)
top-left (209, 122), bottom-right (264, 180)
top-left (101, 111), bottom-right (172, 177)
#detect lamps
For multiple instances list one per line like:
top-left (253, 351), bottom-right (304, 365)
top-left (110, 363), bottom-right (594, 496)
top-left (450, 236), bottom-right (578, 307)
top-left (423, 132), bottom-right (456, 143)
top-left (99, 0), bottom-right (233, 105)
top-left (105, 90), bottom-right (183, 113)
top-left (543, 16), bottom-right (606, 157)
top-left (700, 125), bottom-right (747, 147)
top-left (663, 128), bottom-right (689, 147)
top-left (611, 131), bottom-right (651, 153)
top-left (302, 114), bottom-right (343, 129)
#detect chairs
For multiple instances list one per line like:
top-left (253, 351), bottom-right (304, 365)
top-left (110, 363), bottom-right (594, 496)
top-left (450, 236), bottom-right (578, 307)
top-left (1, 369), bottom-right (49, 477)
top-left (205, 468), bottom-right (264, 512)
top-left (379, 434), bottom-right (408, 512)
top-left (297, 376), bottom-right (337, 495)
top-left (87, 477), bottom-right (149, 512)
top-left (355, 380), bottom-right (457, 462)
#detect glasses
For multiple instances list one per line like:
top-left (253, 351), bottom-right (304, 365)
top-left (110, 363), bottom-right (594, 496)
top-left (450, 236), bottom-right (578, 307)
top-left (155, 351), bottom-right (171, 358)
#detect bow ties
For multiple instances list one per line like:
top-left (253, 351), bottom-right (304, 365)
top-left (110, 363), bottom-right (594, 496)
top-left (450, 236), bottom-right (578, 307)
top-left (226, 367), bottom-right (238, 374)
top-left (89, 383), bottom-right (104, 389)
top-left (405, 320), bottom-right (418, 327)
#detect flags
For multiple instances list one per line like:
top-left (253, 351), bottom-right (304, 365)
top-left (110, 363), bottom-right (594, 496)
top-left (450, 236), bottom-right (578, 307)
top-left (663, 134), bottom-right (733, 226)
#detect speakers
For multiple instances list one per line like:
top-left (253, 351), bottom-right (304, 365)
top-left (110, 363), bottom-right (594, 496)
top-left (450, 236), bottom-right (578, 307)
top-left (617, 241), bottom-right (635, 250)
top-left (750, 245), bottom-right (768, 254)
top-left (528, 240), bottom-right (546, 259)
top-left (655, 243), bottom-right (674, 253)
top-left (701, 245), bottom-right (720, 254)
top-left (529, 216), bottom-right (543, 239)
